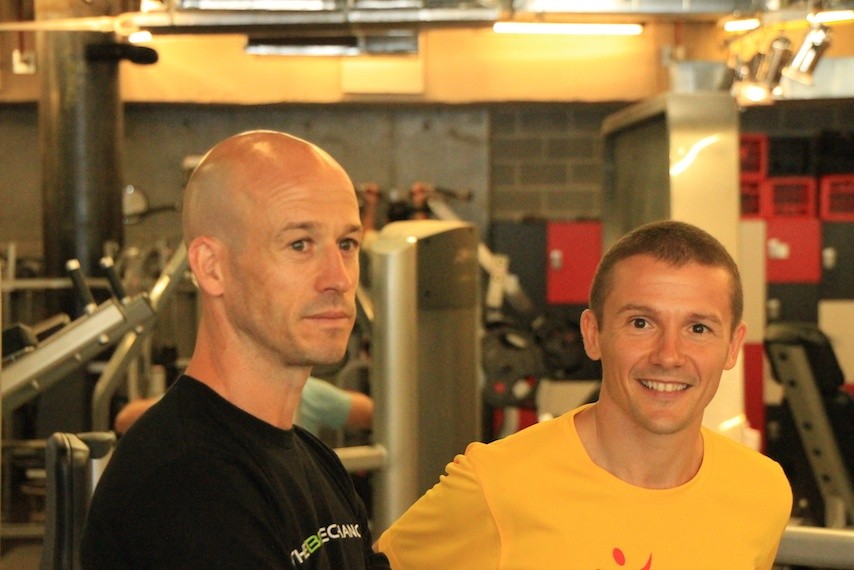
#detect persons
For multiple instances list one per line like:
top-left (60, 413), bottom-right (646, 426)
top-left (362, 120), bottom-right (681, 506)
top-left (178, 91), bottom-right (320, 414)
top-left (369, 219), bottom-right (795, 569)
top-left (355, 180), bottom-right (430, 256)
top-left (115, 375), bottom-right (375, 443)
top-left (77, 125), bottom-right (394, 570)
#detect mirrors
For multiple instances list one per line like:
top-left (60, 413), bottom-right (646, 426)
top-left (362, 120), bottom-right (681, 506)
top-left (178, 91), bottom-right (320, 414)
top-left (124, 185), bottom-right (149, 226)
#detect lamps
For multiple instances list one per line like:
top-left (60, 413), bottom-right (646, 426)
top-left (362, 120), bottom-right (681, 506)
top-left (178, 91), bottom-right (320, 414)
top-left (731, 25), bottom-right (830, 101)
top-left (243, 37), bottom-right (359, 57)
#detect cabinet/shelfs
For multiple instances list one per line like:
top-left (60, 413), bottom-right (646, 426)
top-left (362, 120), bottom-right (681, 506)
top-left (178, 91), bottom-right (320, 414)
top-left (492, 216), bottom-right (601, 331)
